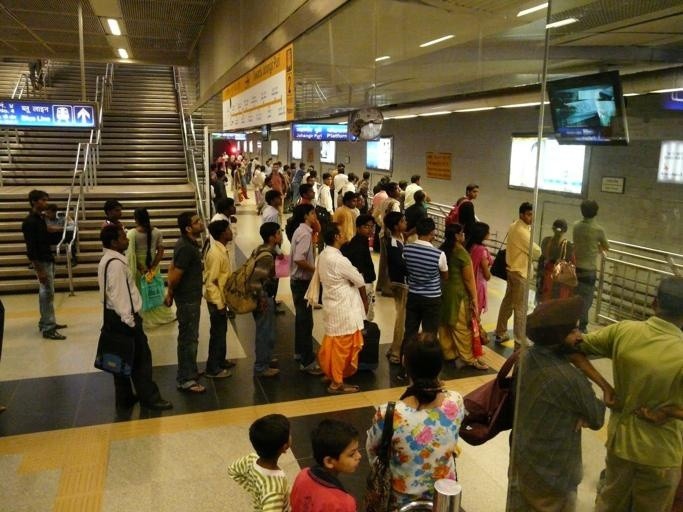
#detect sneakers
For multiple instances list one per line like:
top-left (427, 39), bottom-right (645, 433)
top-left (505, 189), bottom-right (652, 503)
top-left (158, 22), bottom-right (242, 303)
top-left (495, 333), bottom-right (512, 342)
top-left (386, 348), bottom-right (401, 365)
top-left (300, 360), bottom-right (323, 375)
top-left (256, 367), bottom-right (278, 377)
top-left (295, 351), bottom-right (317, 361)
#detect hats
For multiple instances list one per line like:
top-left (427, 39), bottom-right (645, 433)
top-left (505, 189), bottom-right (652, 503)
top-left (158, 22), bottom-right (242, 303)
top-left (525, 296), bottom-right (583, 346)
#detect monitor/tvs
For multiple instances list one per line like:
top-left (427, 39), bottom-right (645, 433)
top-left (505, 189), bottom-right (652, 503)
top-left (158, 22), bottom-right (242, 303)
top-left (545, 70), bottom-right (630, 146)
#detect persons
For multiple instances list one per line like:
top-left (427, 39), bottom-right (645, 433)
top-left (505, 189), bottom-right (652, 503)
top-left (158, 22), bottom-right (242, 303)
top-left (454, 184), bottom-right (477, 230)
top-left (98, 227), bottom-right (173, 410)
top-left (318, 173), bottom-right (334, 218)
top-left (346, 214), bottom-right (376, 323)
top-left (403, 218), bottom-right (448, 370)
top-left (366, 332), bottom-right (463, 512)
top-left (126, 208), bottom-right (175, 328)
top-left (497, 201), bottom-right (541, 354)
top-left (289, 203), bottom-right (321, 375)
top-left (246, 153), bottom-right (295, 194)
top-left (23, 190), bottom-right (68, 340)
top-left (292, 420), bottom-right (360, 511)
top-left (537, 219), bottom-right (574, 316)
top-left (209, 147), bottom-right (251, 204)
top-left (227, 415), bottom-right (291, 511)
top-left (44, 206), bottom-right (78, 267)
top-left (332, 164), bottom-right (369, 220)
top-left (262, 189), bottom-right (281, 225)
top-left (247, 221), bottom-right (282, 377)
top-left (383, 212), bottom-right (406, 363)
top-left (165, 210), bottom-right (205, 394)
top-left (573, 200), bottom-right (608, 329)
top-left (316, 221), bottom-right (367, 393)
top-left (374, 173), bottom-right (405, 212)
top-left (101, 200), bottom-right (124, 235)
top-left (212, 198), bottom-right (235, 226)
top-left (296, 184), bottom-right (322, 260)
top-left (507, 299), bottom-right (604, 511)
top-left (206, 221), bottom-right (236, 379)
top-left (406, 171), bottom-right (426, 193)
top-left (404, 190), bottom-right (425, 237)
top-left (469, 222), bottom-right (492, 361)
top-left (570, 277), bottom-right (681, 511)
top-left (443, 223), bottom-right (488, 369)
top-left (292, 162), bottom-right (320, 187)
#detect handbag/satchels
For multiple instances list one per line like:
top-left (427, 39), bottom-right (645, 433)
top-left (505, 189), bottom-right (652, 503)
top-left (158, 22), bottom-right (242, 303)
top-left (141, 273), bottom-right (166, 311)
top-left (304, 254), bottom-right (324, 308)
top-left (551, 239), bottom-right (579, 284)
top-left (491, 230), bottom-right (509, 281)
top-left (460, 347), bottom-right (527, 446)
top-left (363, 401), bottom-right (394, 511)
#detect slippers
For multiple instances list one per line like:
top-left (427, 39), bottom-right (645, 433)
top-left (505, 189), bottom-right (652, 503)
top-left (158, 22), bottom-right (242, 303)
top-left (329, 383), bottom-right (359, 393)
top-left (207, 358), bottom-right (236, 378)
top-left (178, 382), bottom-right (206, 393)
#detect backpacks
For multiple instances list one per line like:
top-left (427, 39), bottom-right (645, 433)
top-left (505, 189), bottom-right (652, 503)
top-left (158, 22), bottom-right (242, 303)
top-left (444, 199), bottom-right (471, 228)
top-left (223, 249), bottom-right (272, 314)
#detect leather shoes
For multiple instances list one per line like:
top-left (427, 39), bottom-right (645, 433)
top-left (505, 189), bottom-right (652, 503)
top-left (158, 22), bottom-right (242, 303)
top-left (471, 359), bottom-right (490, 370)
top-left (118, 394), bottom-right (138, 410)
top-left (43, 331), bottom-right (67, 340)
top-left (55, 324), bottom-right (68, 328)
top-left (141, 397), bottom-right (173, 409)
